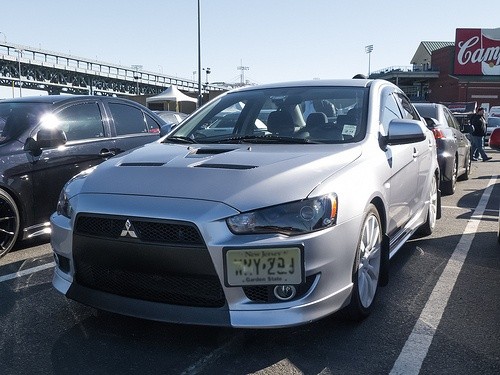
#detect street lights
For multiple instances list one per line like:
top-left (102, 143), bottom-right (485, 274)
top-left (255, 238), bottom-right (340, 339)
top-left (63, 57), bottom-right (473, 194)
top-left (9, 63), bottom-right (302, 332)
top-left (237, 66), bottom-right (249, 84)
top-left (365, 45), bottom-right (374, 75)
top-left (202, 68), bottom-right (211, 81)
top-left (131, 65), bottom-right (143, 95)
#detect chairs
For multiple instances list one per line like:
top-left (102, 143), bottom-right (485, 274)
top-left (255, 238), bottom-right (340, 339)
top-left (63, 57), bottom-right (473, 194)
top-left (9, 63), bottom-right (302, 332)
top-left (267, 110), bottom-right (294, 137)
top-left (295, 112), bottom-right (328, 139)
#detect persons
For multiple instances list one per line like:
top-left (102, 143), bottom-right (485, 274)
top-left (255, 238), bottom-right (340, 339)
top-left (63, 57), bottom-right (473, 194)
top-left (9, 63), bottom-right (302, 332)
top-left (467, 106), bottom-right (492, 162)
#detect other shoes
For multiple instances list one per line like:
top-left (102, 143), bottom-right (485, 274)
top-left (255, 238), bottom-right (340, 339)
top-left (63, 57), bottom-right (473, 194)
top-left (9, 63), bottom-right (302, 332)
top-left (483, 156), bottom-right (492, 162)
top-left (478, 157), bottom-right (482, 160)
top-left (471, 156), bottom-right (478, 161)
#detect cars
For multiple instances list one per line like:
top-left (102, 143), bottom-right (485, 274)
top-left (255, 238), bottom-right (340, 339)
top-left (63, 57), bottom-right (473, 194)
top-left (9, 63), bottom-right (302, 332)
top-left (412, 102), bottom-right (500, 195)
top-left (0, 94), bottom-right (282, 259)
top-left (49, 79), bottom-right (442, 329)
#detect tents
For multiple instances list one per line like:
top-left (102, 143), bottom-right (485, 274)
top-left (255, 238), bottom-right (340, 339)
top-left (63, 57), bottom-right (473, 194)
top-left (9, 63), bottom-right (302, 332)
top-left (146, 86), bottom-right (198, 112)
